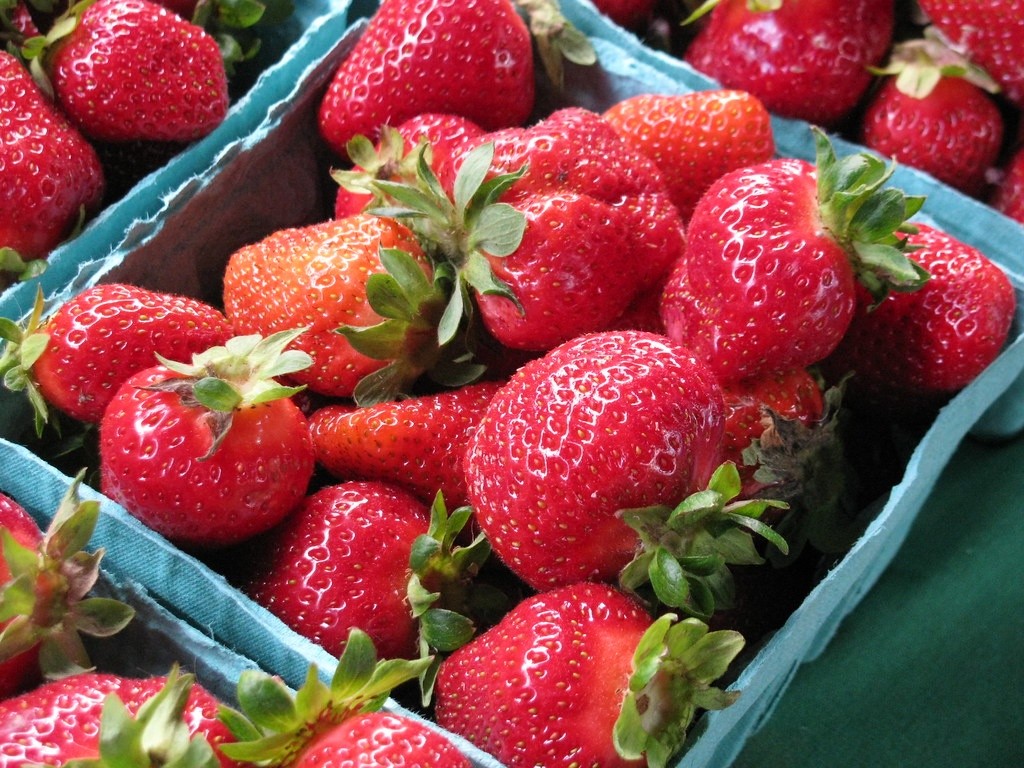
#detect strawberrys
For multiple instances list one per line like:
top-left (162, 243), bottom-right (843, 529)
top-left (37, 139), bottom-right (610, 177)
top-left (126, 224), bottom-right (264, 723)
top-left (0, 0), bottom-right (1024, 768)
